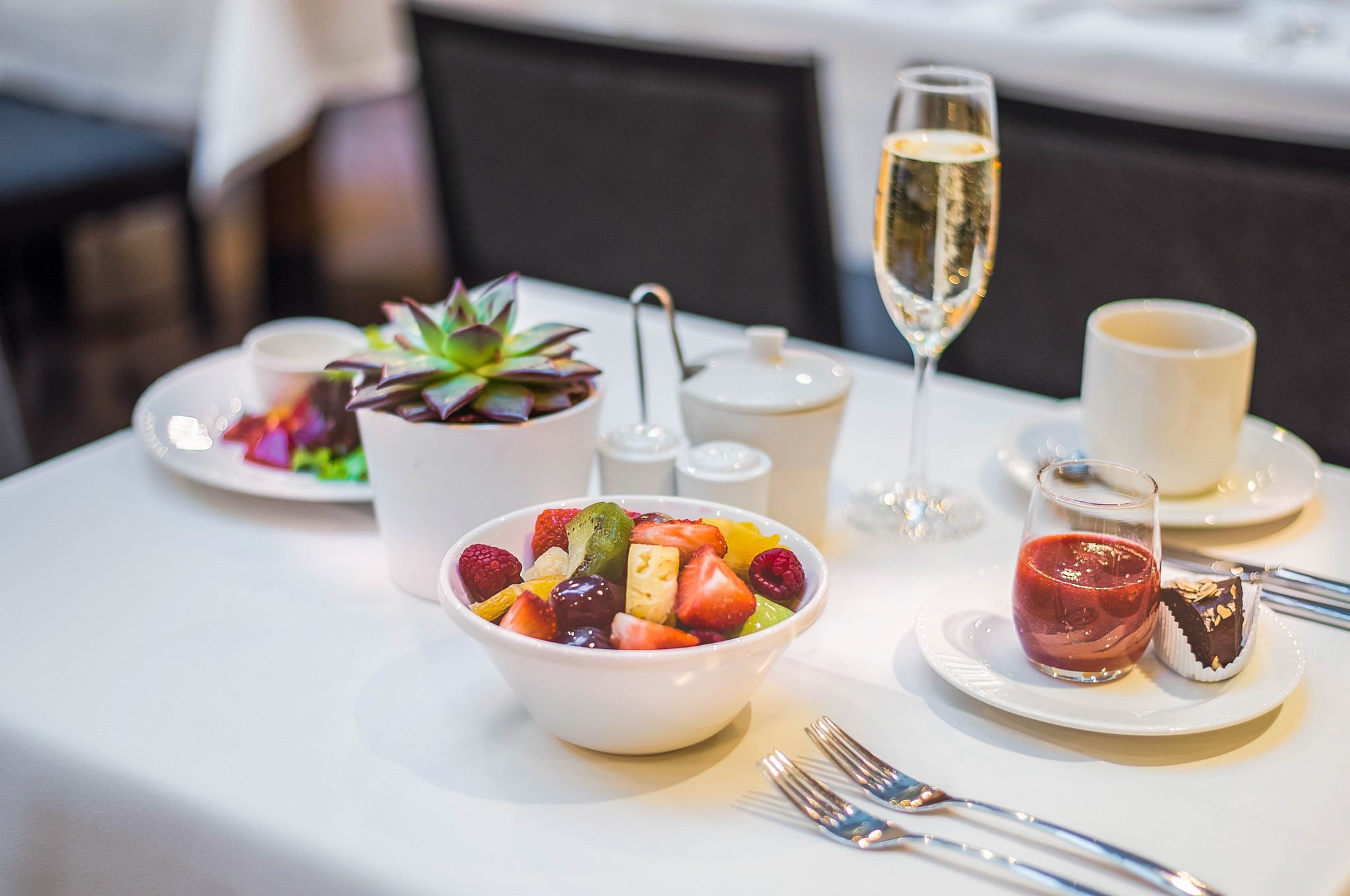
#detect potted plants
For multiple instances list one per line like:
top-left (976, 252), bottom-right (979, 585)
top-left (321, 275), bottom-right (602, 608)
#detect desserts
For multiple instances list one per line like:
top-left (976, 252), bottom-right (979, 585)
top-left (1153, 575), bottom-right (1264, 683)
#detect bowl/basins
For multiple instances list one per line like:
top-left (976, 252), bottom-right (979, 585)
top-left (437, 498), bottom-right (829, 753)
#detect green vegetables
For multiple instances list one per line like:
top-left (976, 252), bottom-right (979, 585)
top-left (289, 443), bottom-right (367, 481)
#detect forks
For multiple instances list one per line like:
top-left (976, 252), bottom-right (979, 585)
top-left (804, 716), bottom-right (1228, 896)
top-left (755, 748), bottom-right (1108, 895)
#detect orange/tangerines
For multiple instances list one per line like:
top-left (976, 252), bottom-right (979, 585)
top-left (697, 516), bottom-right (785, 583)
top-left (471, 574), bottom-right (566, 621)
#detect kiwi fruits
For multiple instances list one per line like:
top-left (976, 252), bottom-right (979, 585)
top-left (566, 502), bottom-right (634, 580)
top-left (719, 594), bottom-right (795, 639)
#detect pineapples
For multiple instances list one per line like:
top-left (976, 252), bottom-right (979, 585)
top-left (623, 542), bottom-right (679, 624)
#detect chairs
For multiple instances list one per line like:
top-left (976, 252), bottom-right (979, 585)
top-left (409, 0), bottom-right (845, 356)
top-left (933, 90), bottom-right (1350, 475)
top-left (1, 88), bottom-right (228, 463)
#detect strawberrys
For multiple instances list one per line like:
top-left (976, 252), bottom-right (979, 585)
top-left (499, 590), bottom-right (559, 642)
top-left (531, 508), bottom-right (584, 557)
top-left (674, 545), bottom-right (756, 630)
top-left (629, 519), bottom-right (728, 562)
top-left (608, 612), bottom-right (700, 649)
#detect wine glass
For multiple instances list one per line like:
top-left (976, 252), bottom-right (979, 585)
top-left (844, 65), bottom-right (1000, 541)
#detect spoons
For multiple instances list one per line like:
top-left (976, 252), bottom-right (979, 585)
top-left (1052, 450), bottom-right (1093, 484)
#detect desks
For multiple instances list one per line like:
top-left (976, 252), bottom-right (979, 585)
top-left (1, 1), bottom-right (1350, 895)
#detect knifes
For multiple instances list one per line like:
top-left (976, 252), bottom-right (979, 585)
top-left (1161, 544), bottom-right (1350, 631)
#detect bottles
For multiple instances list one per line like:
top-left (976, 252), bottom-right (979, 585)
top-left (676, 441), bottom-right (774, 517)
top-left (594, 419), bottom-right (686, 496)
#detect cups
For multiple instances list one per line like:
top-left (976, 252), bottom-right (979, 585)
top-left (1076, 296), bottom-right (1257, 498)
top-left (1010, 464), bottom-right (1161, 685)
top-left (242, 318), bottom-right (364, 408)
top-left (675, 323), bottom-right (853, 547)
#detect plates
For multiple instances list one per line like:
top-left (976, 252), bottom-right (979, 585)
top-left (133, 347), bottom-right (371, 503)
top-left (1000, 397), bottom-right (1324, 529)
top-left (916, 565), bottom-right (1306, 736)
